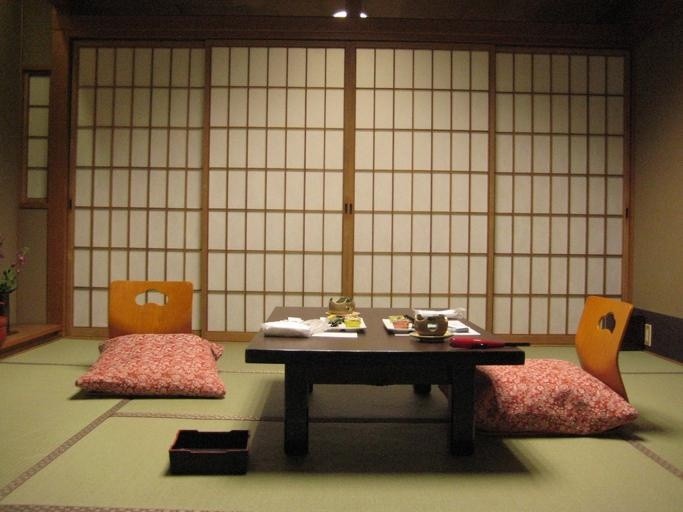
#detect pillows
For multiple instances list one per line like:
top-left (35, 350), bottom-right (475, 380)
top-left (435, 356), bottom-right (641, 439)
top-left (73, 333), bottom-right (230, 401)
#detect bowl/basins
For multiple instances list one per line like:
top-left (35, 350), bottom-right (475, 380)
top-left (416, 312), bottom-right (448, 337)
top-left (328, 296), bottom-right (354, 315)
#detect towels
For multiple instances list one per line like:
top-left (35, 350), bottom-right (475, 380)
top-left (412, 308), bottom-right (460, 321)
top-left (259, 315), bottom-right (310, 340)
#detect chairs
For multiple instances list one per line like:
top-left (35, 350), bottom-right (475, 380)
top-left (574, 294), bottom-right (636, 404)
top-left (107, 280), bottom-right (194, 340)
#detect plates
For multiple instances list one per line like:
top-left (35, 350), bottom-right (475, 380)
top-left (381, 318), bottom-right (415, 333)
top-left (408, 331), bottom-right (452, 342)
top-left (324, 310), bottom-right (359, 317)
top-left (319, 315), bottom-right (367, 332)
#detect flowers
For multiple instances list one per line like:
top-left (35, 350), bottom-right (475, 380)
top-left (0, 241), bottom-right (33, 315)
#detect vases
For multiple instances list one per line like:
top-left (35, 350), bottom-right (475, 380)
top-left (0, 316), bottom-right (8, 342)
